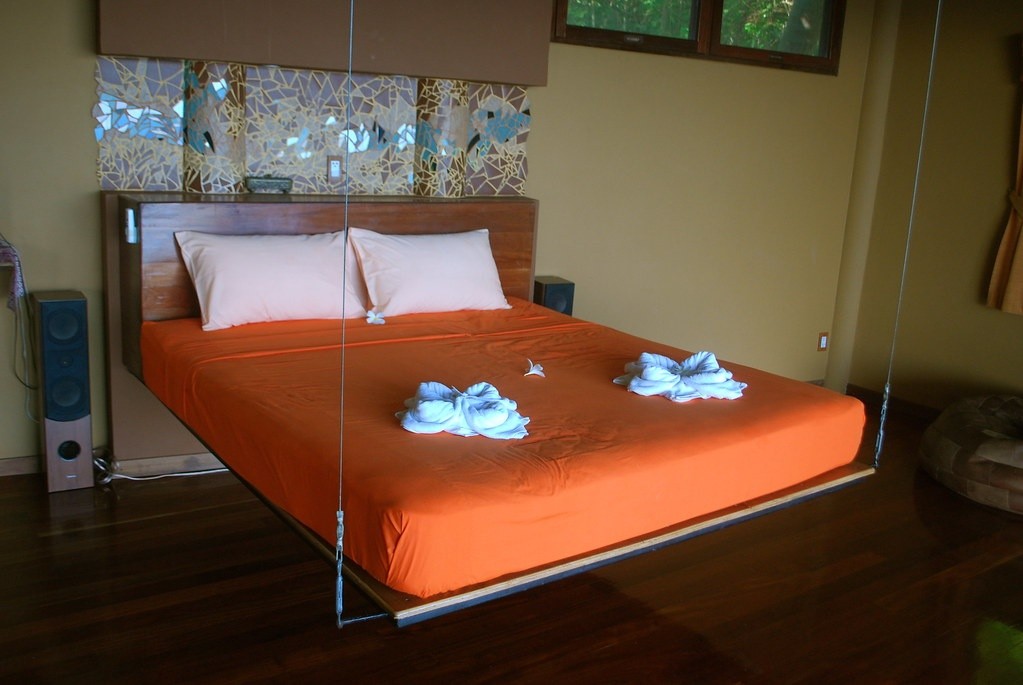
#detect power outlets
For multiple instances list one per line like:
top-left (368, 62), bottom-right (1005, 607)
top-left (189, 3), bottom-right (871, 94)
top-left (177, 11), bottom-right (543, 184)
top-left (328, 156), bottom-right (342, 181)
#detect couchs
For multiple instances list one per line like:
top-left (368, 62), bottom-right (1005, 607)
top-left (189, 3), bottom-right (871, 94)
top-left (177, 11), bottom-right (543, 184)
top-left (918, 394), bottom-right (1023, 515)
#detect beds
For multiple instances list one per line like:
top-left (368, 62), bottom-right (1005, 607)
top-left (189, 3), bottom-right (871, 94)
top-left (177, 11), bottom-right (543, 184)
top-left (99, 190), bottom-right (877, 628)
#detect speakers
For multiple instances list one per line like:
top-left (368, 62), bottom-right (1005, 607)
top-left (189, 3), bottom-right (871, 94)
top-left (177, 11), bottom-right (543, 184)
top-left (534, 276), bottom-right (575, 316)
top-left (29, 290), bottom-right (94, 492)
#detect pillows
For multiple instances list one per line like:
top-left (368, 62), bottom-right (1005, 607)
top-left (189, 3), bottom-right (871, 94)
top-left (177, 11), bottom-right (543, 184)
top-left (348, 227), bottom-right (513, 319)
top-left (173, 230), bottom-right (372, 332)
